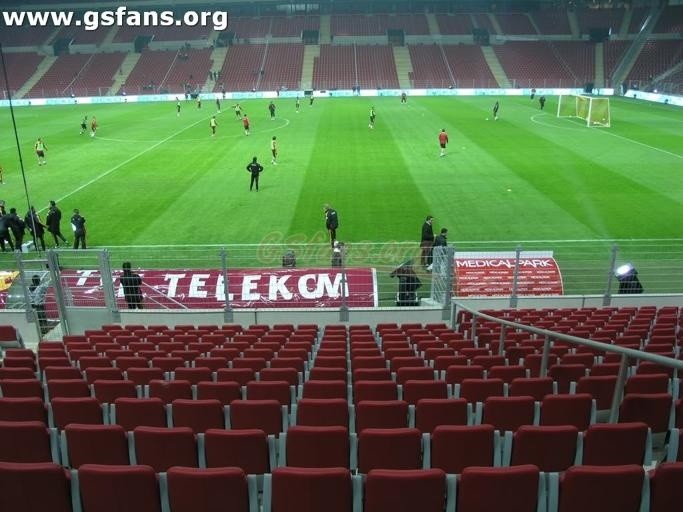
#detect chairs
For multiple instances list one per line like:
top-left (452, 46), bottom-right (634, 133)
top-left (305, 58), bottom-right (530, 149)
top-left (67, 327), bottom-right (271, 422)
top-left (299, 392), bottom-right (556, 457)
top-left (0, 305), bottom-right (683, 512)
top-left (0, 9), bottom-right (683, 98)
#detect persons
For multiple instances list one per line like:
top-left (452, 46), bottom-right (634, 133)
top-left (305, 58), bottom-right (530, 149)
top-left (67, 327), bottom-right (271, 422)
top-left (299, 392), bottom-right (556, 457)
top-left (439, 128), bottom-right (448, 156)
top-left (332, 242), bottom-right (345, 267)
top-left (368, 106), bottom-right (376, 129)
top-left (401, 91), bottom-right (407, 105)
top-left (493, 101), bottom-right (499, 120)
top-left (539, 95), bottom-right (546, 110)
top-left (119, 64), bottom-right (123, 75)
top-left (530, 87), bottom-right (536, 99)
top-left (321, 204), bottom-right (339, 248)
top-left (269, 101), bottom-right (276, 120)
top-left (352, 84), bottom-right (361, 96)
top-left (119, 262), bottom-right (144, 309)
top-left (276, 86), bottom-right (280, 97)
top-left (246, 157), bottom-right (264, 192)
top-left (80, 115), bottom-right (99, 137)
top-left (271, 136), bottom-right (278, 165)
top-left (310, 94), bottom-right (314, 106)
top-left (35, 138), bottom-right (48, 165)
top-left (295, 97), bottom-right (300, 113)
top-left (142, 36), bottom-right (265, 136)
top-left (0, 199), bottom-right (87, 254)
top-left (29, 271), bottom-right (61, 336)
top-left (419, 215), bottom-right (447, 272)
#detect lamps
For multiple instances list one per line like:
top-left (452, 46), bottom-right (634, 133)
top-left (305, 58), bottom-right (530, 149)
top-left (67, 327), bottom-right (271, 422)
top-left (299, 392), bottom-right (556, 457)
top-left (614, 263), bottom-right (643, 293)
top-left (390, 259), bottom-right (422, 306)
